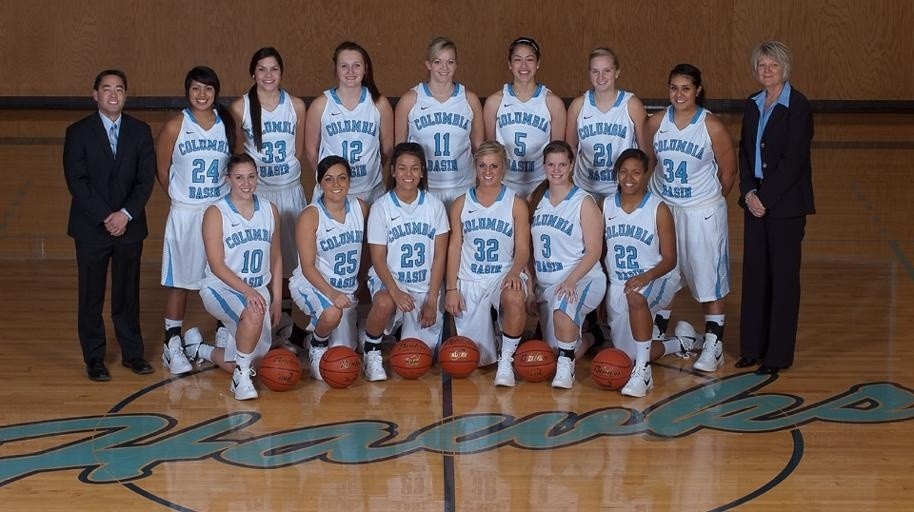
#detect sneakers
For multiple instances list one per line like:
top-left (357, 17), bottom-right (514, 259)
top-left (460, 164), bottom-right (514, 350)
top-left (551, 356), bottom-right (577, 390)
top-left (228, 364), bottom-right (258, 402)
top-left (162, 327), bottom-right (203, 375)
top-left (495, 356), bottom-right (516, 387)
top-left (308, 344), bottom-right (328, 382)
top-left (362, 348), bottom-right (387, 382)
top-left (621, 362), bottom-right (653, 398)
top-left (213, 328), bottom-right (229, 349)
top-left (675, 320), bottom-right (725, 372)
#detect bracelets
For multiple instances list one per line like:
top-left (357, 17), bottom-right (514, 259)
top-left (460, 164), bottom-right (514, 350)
top-left (446, 288), bottom-right (457, 291)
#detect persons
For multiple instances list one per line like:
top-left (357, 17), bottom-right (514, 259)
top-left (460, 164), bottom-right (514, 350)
top-left (156, 67), bottom-right (236, 376)
top-left (601, 148), bottom-right (704, 397)
top-left (484, 38), bottom-right (568, 199)
top-left (182, 153), bottom-right (282, 399)
top-left (643, 65), bottom-right (734, 370)
top-left (230, 49), bottom-right (306, 352)
top-left (62, 69), bottom-right (154, 382)
top-left (737, 39), bottom-right (813, 376)
top-left (444, 141), bottom-right (530, 387)
top-left (304, 45), bottom-right (395, 206)
top-left (393, 38), bottom-right (483, 338)
top-left (364, 143), bottom-right (450, 381)
top-left (567, 47), bottom-right (646, 341)
top-left (289, 157), bottom-right (369, 379)
top-left (526, 141), bottom-right (607, 389)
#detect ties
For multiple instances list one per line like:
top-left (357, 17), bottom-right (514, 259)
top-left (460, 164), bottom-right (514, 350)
top-left (109, 124), bottom-right (117, 157)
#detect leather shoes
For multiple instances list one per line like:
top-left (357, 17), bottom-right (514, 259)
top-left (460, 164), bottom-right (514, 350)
top-left (755, 363), bottom-right (792, 375)
top-left (734, 356), bottom-right (758, 368)
top-left (86, 361), bottom-right (111, 382)
top-left (121, 357), bottom-right (153, 375)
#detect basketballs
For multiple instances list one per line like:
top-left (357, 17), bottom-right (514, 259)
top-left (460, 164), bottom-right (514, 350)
top-left (515, 339), bottom-right (556, 383)
top-left (437, 336), bottom-right (482, 379)
top-left (590, 348), bottom-right (633, 390)
top-left (389, 337), bottom-right (432, 380)
top-left (319, 345), bottom-right (361, 387)
top-left (260, 349), bottom-right (303, 390)
